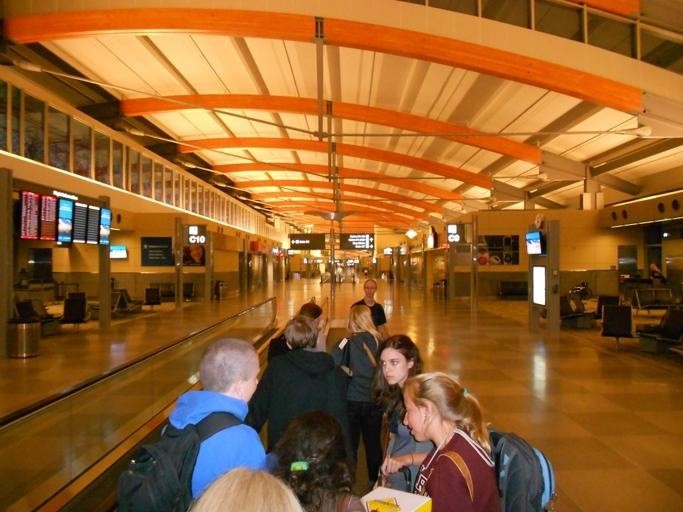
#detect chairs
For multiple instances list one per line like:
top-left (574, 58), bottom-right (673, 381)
top-left (15, 291), bottom-right (88, 331)
top-left (111, 283), bottom-right (176, 318)
top-left (556, 287), bottom-right (683, 354)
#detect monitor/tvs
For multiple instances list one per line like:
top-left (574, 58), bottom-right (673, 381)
top-left (525, 231), bottom-right (543, 255)
top-left (109, 245), bottom-right (128, 259)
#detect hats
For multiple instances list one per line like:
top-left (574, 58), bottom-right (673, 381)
top-left (300, 304), bottom-right (323, 320)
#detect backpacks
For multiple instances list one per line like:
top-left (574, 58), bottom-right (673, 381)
top-left (118, 412), bottom-right (244, 512)
top-left (490, 432), bottom-right (555, 512)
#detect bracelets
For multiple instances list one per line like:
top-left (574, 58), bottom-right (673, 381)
top-left (407, 451), bottom-right (414, 467)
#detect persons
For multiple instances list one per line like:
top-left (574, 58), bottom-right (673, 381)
top-left (182, 245), bottom-right (195, 265)
top-left (187, 464), bottom-right (305, 512)
top-left (266, 301), bottom-right (332, 363)
top-left (370, 333), bottom-right (436, 494)
top-left (401, 371), bottom-right (502, 511)
top-left (194, 244), bottom-right (205, 265)
top-left (346, 277), bottom-right (391, 339)
top-left (240, 313), bottom-right (352, 482)
top-left (270, 408), bottom-right (367, 511)
top-left (159, 336), bottom-right (271, 500)
top-left (649, 263), bottom-right (667, 284)
top-left (332, 304), bottom-right (385, 490)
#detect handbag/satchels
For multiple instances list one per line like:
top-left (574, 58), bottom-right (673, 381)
top-left (337, 360), bottom-right (353, 393)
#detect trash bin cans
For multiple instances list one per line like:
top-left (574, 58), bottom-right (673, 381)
top-left (434, 282), bottom-right (440, 299)
top-left (8, 319), bottom-right (40, 358)
top-left (217, 281), bottom-right (224, 300)
top-left (441, 280), bottom-right (446, 300)
